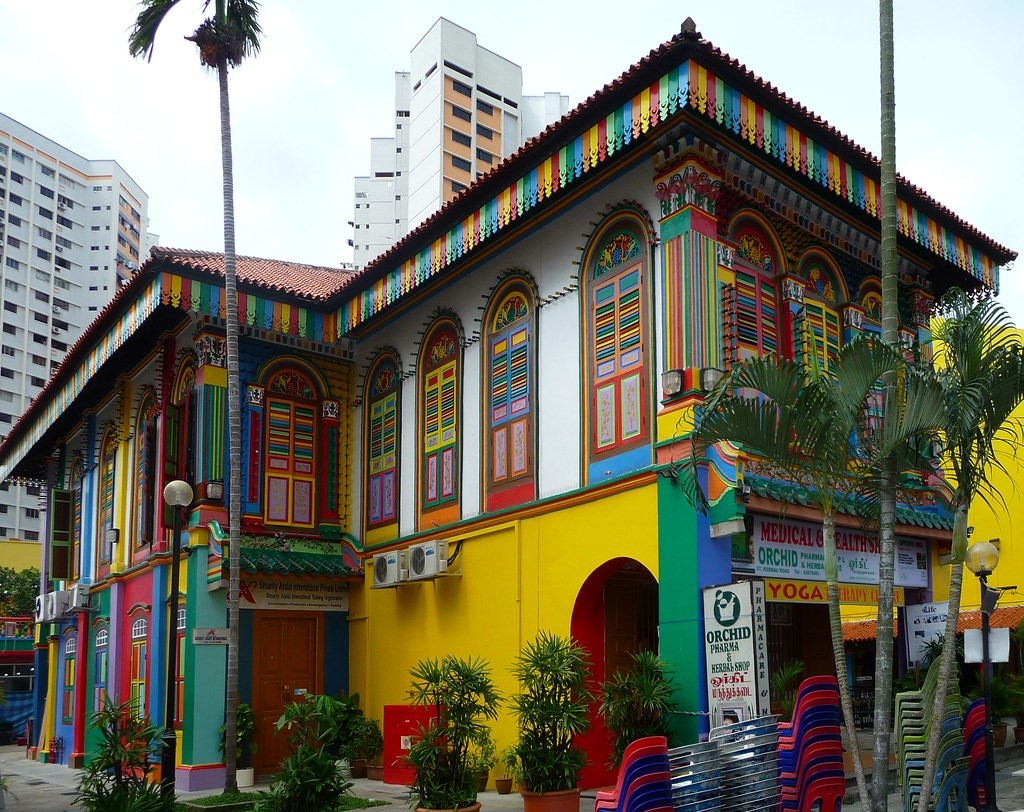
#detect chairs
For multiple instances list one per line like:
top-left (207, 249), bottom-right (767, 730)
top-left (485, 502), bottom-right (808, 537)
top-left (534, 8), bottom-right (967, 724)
top-left (893, 652), bottom-right (998, 812)
top-left (594, 675), bottom-right (846, 812)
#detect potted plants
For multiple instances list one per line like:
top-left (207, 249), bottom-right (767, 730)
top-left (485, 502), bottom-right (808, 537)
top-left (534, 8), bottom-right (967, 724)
top-left (273, 688), bottom-right (384, 780)
top-left (218, 702), bottom-right (256, 788)
top-left (390, 634), bottom-right (597, 812)
top-left (968, 668), bottom-right (1024, 748)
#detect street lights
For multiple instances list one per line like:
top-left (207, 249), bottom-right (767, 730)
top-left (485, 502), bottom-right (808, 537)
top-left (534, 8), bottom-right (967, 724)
top-left (161, 479), bottom-right (193, 812)
top-left (967, 540), bottom-right (1005, 811)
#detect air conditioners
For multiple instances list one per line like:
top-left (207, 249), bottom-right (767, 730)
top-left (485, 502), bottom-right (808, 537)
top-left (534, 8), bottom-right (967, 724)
top-left (35, 594), bottom-right (56, 624)
top-left (47, 591), bottom-right (80, 622)
top-left (64, 583), bottom-right (101, 614)
top-left (408, 540), bottom-right (463, 580)
top-left (373, 549), bottom-right (421, 589)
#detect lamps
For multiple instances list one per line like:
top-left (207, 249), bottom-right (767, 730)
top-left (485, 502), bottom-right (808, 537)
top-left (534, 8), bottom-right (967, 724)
top-left (205, 480), bottom-right (223, 500)
top-left (105, 529), bottom-right (118, 544)
top-left (700, 368), bottom-right (725, 392)
top-left (661, 368), bottom-right (683, 396)
